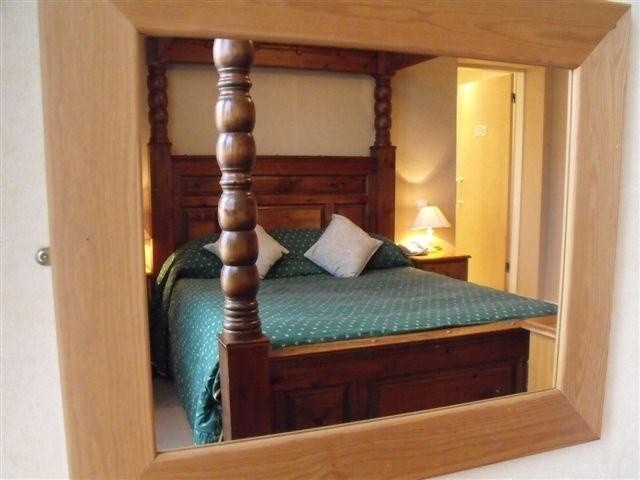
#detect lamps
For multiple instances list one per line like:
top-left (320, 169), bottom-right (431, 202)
top-left (415, 208), bottom-right (451, 250)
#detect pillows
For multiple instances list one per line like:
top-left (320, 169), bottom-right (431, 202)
top-left (178, 229), bottom-right (405, 277)
top-left (302, 215), bottom-right (382, 278)
top-left (205, 218), bottom-right (291, 280)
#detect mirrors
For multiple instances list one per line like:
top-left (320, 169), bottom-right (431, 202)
top-left (34, 2), bottom-right (630, 479)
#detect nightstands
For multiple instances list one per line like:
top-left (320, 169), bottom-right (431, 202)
top-left (403, 249), bottom-right (472, 282)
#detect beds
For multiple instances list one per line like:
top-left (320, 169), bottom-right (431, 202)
top-left (148, 40), bottom-right (558, 442)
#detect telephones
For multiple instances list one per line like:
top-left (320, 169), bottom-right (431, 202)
top-left (398, 240), bottom-right (428, 255)
